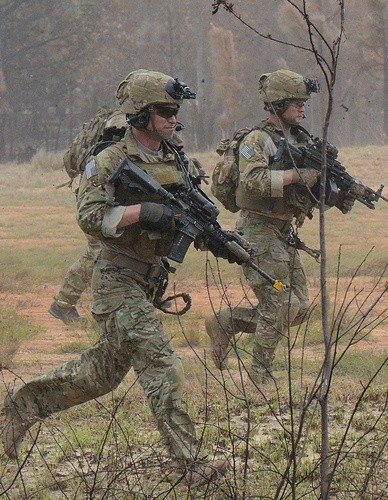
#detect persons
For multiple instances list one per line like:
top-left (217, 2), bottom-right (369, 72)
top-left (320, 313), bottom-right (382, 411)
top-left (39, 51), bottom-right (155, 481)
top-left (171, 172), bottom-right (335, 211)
top-left (4, 68), bottom-right (253, 492)
top-left (204, 69), bottom-right (367, 391)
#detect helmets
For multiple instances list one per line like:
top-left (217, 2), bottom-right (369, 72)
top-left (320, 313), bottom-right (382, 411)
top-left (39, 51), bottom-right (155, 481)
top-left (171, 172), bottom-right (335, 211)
top-left (115, 68), bottom-right (183, 115)
top-left (259, 70), bottom-right (311, 104)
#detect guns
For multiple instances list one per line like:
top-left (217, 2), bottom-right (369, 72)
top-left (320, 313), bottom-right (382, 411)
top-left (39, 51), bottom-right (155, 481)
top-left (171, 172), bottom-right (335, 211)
top-left (274, 136), bottom-right (388, 211)
top-left (106, 157), bottom-right (289, 295)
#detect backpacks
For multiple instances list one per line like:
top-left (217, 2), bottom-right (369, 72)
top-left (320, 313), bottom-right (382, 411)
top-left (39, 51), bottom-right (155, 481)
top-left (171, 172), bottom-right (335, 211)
top-left (63, 105), bottom-right (122, 179)
top-left (210, 127), bottom-right (289, 212)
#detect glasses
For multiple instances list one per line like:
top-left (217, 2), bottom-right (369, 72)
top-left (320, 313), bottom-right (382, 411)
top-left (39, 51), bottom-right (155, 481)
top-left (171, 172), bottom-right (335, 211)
top-left (289, 98), bottom-right (308, 107)
top-left (154, 104), bottom-right (179, 118)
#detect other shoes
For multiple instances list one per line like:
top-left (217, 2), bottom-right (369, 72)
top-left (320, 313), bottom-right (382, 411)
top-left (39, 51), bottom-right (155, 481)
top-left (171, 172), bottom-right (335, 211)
top-left (156, 299), bottom-right (171, 308)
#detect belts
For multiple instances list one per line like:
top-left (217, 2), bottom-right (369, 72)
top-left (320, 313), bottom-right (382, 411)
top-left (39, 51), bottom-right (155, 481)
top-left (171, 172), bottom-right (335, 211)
top-left (100, 249), bottom-right (150, 276)
top-left (240, 210), bottom-right (285, 230)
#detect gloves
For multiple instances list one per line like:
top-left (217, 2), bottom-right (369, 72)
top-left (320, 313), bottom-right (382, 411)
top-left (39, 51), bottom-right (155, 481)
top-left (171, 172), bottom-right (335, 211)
top-left (291, 166), bottom-right (322, 189)
top-left (138, 201), bottom-right (176, 235)
top-left (335, 190), bottom-right (358, 215)
top-left (210, 229), bottom-right (253, 265)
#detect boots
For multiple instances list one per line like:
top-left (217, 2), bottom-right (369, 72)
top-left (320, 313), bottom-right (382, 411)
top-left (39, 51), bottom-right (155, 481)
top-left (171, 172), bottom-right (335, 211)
top-left (246, 344), bottom-right (282, 388)
top-left (205, 314), bottom-right (230, 370)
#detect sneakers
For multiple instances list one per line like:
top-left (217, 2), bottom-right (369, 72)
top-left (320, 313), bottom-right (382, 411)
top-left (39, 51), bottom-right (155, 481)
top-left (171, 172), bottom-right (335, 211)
top-left (49, 302), bottom-right (89, 327)
top-left (175, 458), bottom-right (230, 492)
top-left (4, 382), bottom-right (31, 460)
